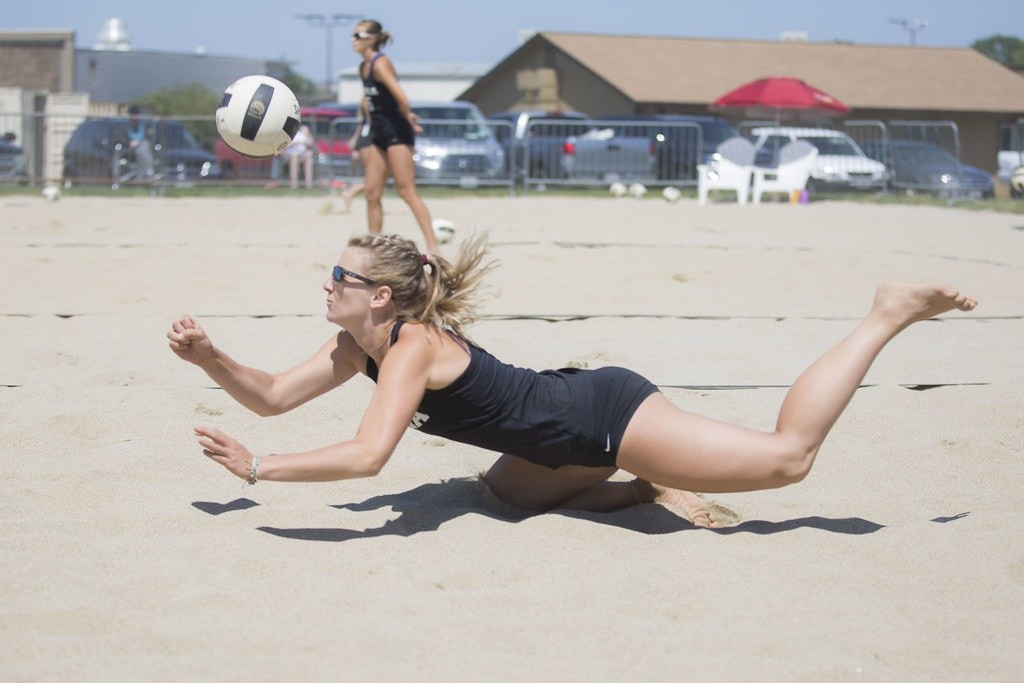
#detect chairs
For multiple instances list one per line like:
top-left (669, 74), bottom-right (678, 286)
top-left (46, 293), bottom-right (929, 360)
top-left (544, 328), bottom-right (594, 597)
top-left (752, 138), bottom-right (821, 204)
top-left (696, 135), bottom-right (757, 206)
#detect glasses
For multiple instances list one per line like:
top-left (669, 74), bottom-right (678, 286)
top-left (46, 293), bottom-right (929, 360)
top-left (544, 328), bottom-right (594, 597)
top-left (332, 265), bottom-right (396, 303)
top-left (352, 32), bottom-right (373, 39)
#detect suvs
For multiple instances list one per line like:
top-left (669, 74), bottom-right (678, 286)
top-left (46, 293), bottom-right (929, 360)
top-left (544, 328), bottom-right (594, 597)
top-left (62, 117), bottom-right (225, 189)
top-left (209, 109), bottom-right (363, 180)
top-left (858, 139), bottom-right (994, 197)
top-left (640, 116), bottom-right (778, 181)
top-left (749, 128), bottom-right (890, 192)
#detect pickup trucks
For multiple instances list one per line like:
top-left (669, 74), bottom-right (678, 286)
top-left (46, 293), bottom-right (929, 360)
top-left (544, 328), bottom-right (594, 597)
top-left (491, 109), bottom-right (657, 180)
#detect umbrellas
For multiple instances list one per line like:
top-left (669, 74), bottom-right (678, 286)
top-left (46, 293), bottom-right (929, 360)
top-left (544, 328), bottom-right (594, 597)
top-left (710, 76), bottom-right (852, 201)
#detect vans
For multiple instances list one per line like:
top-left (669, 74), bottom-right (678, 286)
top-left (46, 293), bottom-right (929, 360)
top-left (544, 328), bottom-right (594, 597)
top-left (408, 101), bottom-right (508, 181)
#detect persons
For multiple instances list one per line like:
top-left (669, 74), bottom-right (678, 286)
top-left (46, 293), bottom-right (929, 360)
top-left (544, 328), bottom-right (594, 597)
top-left (346, 20), bottom-right (441, 252)
top-left (114, 105), bottom-right (156, 178)
top-left (281, 125), bottom-right (313, 187)
top-left (165, 235), bottom-right (978, 525)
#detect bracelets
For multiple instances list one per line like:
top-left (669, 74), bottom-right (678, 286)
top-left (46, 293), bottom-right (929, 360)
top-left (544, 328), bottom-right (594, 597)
top-left (248, 456), bottom-right (260, 484)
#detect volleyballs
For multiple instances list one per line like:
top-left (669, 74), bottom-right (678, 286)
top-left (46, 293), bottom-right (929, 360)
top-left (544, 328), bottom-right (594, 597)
top-left (215, 76), bottom-right (301, 156)
